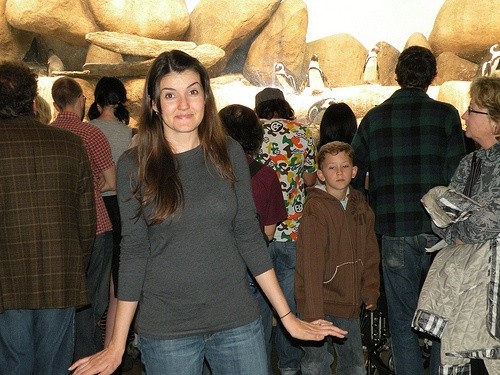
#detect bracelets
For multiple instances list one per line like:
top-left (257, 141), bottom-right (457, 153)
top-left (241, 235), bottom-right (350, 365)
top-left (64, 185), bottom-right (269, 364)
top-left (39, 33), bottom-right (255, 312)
top-left (277, 311), bottom-right (292, 319)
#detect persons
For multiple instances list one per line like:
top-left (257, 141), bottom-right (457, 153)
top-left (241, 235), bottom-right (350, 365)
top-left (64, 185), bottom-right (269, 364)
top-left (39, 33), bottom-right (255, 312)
top-left (47, 78), bottom-right (117, 363)
top-left (252, 88), bottom-right (318, 375)
top-left (294, 140), bottom-right (381, 375)
top-left (68, 49), bottom-right (347, 375)
top-left (316, 103), bottom-right (358, 150)
top-left (350, 45), bottom-right (465, 375)
top-left (87, 75), bottom-right (138, 299)
top-left (0, 60), bottom-right (98, 375)
top-left (36, 96), bottom-right (52, 124)
top-left (411, 78), bottom-right (500, 375)
top-left (218, 104), bottom-right (289, 246)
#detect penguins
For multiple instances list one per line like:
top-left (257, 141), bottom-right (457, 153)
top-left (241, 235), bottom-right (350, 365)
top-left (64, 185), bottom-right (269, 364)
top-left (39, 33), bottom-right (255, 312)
top-left (47, 48), bottom-right (64, 76)
top-left (472, 43), bottom-right (500, 78)
top-left (274, 63), bottom-right (301, 96)
top-left (359, 46), bottom-right (380, 85)
top-left (301, 54), bottom-right (332, 95)
top-left (308, 98), bottom-right (337, 131)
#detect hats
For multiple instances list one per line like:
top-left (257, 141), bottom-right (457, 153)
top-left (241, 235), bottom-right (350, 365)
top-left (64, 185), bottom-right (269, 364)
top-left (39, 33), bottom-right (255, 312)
top-left (255, 88), bottom-right (285, 107)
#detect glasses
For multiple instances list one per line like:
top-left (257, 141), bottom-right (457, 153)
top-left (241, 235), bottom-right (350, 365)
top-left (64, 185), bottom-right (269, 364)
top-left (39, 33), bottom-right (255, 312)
top-left (468, 106), bottom-right (490, 115)
